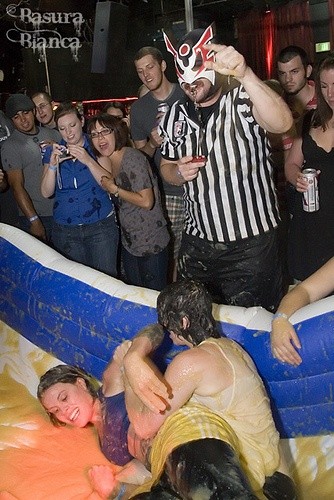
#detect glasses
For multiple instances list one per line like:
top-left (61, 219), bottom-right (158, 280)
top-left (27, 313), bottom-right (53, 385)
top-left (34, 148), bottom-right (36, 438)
top-left (87, 125), bottom-right (114, 138)
top-left (34, 101), bottom-right (51, 112)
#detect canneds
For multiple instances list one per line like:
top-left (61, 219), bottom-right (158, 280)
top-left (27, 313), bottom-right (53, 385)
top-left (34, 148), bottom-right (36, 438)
top-left (301, 169), bottom-right (321, 212)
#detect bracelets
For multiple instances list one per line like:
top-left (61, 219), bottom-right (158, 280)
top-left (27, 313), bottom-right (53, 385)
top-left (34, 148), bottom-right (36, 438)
top-left (28, 215), bottom-right (39, 223)
top-left (115, 187), bottom-right (121, 198)
top-left (48, 162), bottom-right (59, 169)
top-left (175, 165), bottom-right (187, 182)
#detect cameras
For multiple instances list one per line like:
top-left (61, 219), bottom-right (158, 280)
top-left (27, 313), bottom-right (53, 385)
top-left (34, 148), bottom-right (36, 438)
top-left (59, 148), bottom-right (73, 157)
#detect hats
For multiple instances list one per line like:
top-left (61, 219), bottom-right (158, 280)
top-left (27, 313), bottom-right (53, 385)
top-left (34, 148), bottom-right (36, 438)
top-left (3, 94), bottom-right (34, 118)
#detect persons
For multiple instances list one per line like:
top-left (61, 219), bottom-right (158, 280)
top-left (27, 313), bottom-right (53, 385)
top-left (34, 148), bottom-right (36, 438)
top-left (1, 92), bottom-right (64, 246)
top-left (112, 276), bottom-right (298, 500)
top-left (270, 256), bottom-right (334, 366)
top-left (129, 46), bottom-right (190, 284)
top-left (36, 338), bottom-right (266, 500)
top-left (105, 101), bottom-right (131, 137)
top-left (156, 29), bottom-right (294, 318)
top-left (86, 113), bottom-right (172, 293)
top-left (269, 44), bottom-right (321, 228)
top-left (283, 56), bottom-right (334, 276)
top-left (29, 91), bottom-right (59, 132)
top-left (263, 78), bottom-right (284, 99)
top-left (1, 108), bottom-right (19, 225)
top-left (137, 85), bottom-right (150, 96)
top-left (40, 104), bottom-right (120, 279)
